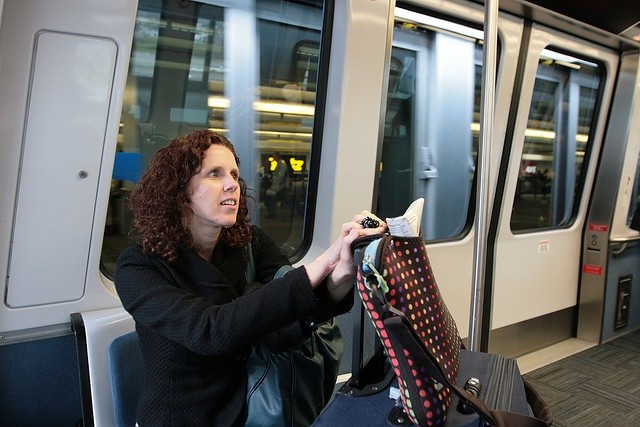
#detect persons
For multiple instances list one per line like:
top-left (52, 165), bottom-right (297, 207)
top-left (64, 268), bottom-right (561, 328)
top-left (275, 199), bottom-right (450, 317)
top-left (114, 129), bottom-right (384, 427)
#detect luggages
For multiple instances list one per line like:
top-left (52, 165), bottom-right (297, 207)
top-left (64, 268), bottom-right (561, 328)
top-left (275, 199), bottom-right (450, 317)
top-left (307, 236), bottom-right (549, 427)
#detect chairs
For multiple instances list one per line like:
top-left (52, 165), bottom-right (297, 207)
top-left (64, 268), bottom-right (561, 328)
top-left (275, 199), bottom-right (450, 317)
top-left (72, 304), bottom-right (150, 427)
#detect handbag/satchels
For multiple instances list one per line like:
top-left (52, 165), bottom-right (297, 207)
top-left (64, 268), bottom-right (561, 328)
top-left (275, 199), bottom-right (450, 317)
top-left (243, 267), bottom-right (343, 427)
top-left (358, 232), bottom-right (461, 427)
